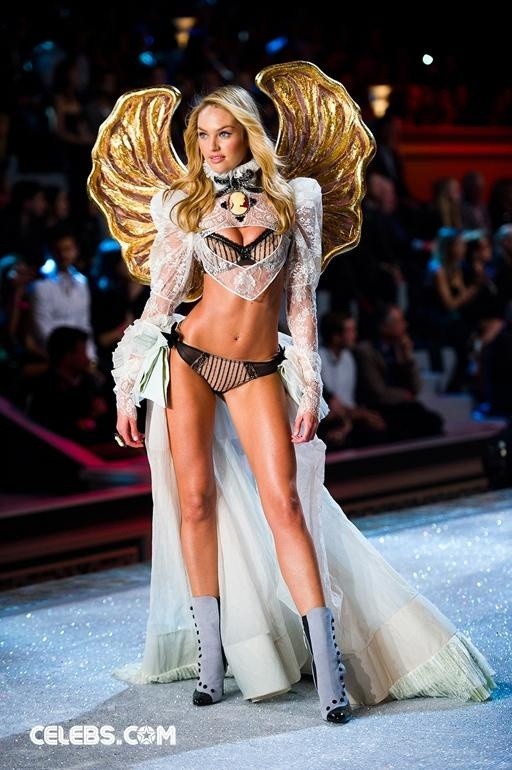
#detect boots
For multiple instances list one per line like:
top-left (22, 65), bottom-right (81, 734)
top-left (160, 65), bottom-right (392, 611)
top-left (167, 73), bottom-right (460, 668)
top-left (189, 594), bottom-right (229, 706)
top-left (298, 606), bottom-right (352, 724)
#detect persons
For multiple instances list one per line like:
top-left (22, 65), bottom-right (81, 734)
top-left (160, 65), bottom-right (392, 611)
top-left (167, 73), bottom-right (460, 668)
top-left (106, 57), bottom-right (354, 726)
top-left (1, 2), bottom-right (512, 465)
